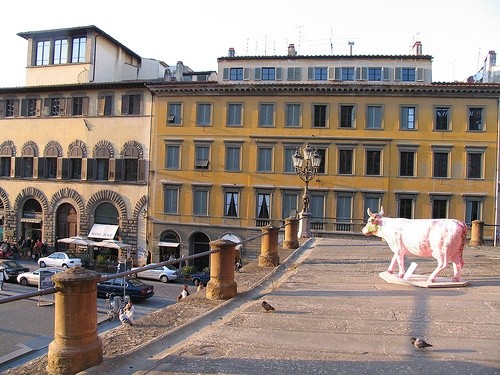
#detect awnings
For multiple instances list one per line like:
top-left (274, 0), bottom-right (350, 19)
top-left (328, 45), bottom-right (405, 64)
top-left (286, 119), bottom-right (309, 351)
top-left (88, 224), bottom-right (119, 240)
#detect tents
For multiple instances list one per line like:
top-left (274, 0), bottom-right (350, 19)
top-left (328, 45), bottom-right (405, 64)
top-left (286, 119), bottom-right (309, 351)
top-left (88, 238), bottom-right (132, 263)
top-left (57, 235), bottom-right (95, 257)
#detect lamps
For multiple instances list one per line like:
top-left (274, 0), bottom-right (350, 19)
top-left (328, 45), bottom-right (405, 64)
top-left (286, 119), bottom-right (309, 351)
top-left (293, 148), bottom-right (320, 212)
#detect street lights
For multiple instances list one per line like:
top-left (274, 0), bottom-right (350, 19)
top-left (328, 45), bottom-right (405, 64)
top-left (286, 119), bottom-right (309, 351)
top-left (292, 141), bottom-right (321, 213)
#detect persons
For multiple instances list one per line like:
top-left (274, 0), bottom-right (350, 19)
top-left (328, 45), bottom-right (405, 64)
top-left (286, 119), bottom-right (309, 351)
top-left (0, 262), bottom-right (9, 290)
top-left (161, 252), bottom-right (186, 269)
top-left (180, 284), bottom-right (190, 300)
top-left (236, 259), bottom-right (242, 272)
top-left (196, 282), bottom-right (204, 292)
top-left (146, 250), bottom-right (151, 265)
top-left (123, 300), bottom-right (134, 321)
top-left (10, 236), bottom-right (48, 262)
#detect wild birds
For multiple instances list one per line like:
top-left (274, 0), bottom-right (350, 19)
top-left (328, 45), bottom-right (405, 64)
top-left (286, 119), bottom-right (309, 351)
top-left (234, 258), bottom-right (244, 273)
top-left (409, 336), bottom-right (434, 352)
top-left (260, 300), bottom-right (276, 314)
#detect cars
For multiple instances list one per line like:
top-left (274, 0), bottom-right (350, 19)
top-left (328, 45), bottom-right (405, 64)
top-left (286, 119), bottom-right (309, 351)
top-left (190, 269), bottom-right (210, 286)
top-left (38, 252), bottom-right (83, 268)
top-left (129, 262), bottom-right (183, 283)
top-left (0, 259), bottom-right (30, 282)
top-left (16, 267), bottom-right (68, 286)
top-left (97, 274), bottom-right (155, 304)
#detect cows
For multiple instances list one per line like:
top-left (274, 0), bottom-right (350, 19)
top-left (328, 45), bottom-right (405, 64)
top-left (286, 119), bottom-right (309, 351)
top-left (360, 204), bottom-right (468, 284)
